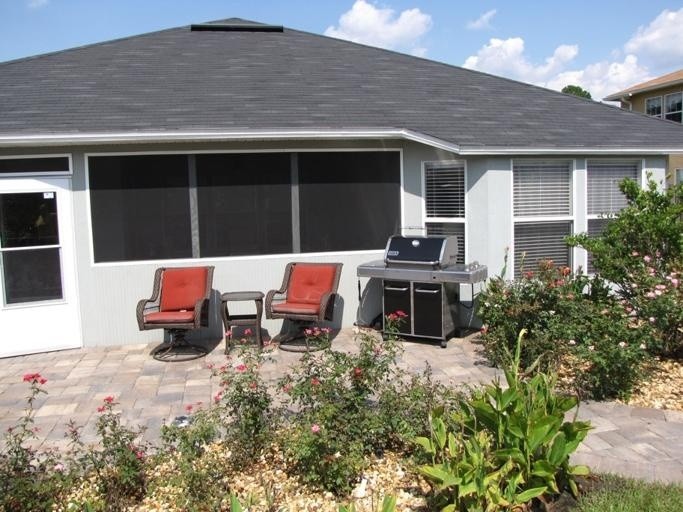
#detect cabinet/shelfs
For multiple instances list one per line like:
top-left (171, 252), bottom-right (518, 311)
top-left (381, 278), bottom-right (461, 348)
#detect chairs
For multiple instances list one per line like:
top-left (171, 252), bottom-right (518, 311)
top-left (136, 263), bottom-right (343, 364)
